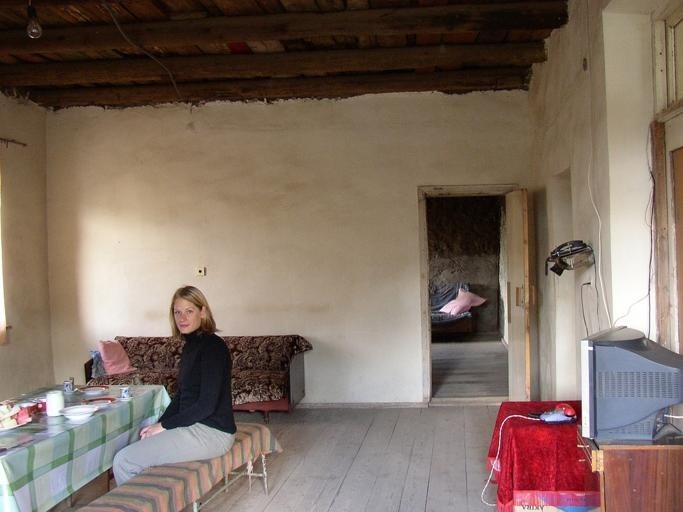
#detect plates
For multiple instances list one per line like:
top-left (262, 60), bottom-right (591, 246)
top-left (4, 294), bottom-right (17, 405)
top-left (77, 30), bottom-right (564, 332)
top-left (78, 385), bottom-right (118, 407)
top-left (117, 396), bottom-right (135, 403)
top-left (62, 389), bottom-right (75, 395)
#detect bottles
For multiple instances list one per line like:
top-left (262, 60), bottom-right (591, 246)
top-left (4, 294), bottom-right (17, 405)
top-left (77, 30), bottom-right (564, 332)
top-left (45, 391), bottom-right (64, 416)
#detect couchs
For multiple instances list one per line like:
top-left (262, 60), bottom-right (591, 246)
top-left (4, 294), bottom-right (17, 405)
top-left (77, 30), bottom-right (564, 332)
top-left (429, 282), bottom-right (472, 341)
top-left (83, 336), bottom-right (315, 425)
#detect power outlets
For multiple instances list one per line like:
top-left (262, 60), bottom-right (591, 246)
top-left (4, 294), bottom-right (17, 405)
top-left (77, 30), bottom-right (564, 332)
top-left (582, 272), bottom-right (594, 287)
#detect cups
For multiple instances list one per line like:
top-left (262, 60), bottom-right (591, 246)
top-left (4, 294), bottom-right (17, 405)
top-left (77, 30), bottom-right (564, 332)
top-left (64, 381), bottom-right (72, 390)
top-left (121, 387), bottom-right (130, 397)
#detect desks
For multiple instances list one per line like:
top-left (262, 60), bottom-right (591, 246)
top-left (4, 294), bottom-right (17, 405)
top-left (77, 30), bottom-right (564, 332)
top-left (573, 423), bottom-right (683, 512)
top-left (486, 400), bottom-right (602, 511)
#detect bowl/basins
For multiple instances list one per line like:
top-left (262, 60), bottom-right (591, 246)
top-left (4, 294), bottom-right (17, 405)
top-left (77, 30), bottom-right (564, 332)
top-left (59, 405), bottom-right (98, 422)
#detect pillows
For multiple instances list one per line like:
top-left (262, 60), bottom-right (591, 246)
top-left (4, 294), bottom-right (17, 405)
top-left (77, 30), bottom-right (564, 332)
top-left (438, 288), bottom-right (488, 317)
top-left (89, 351), bottom-right (106, 378)
top-left (95, 339), bottom-right (137, 376)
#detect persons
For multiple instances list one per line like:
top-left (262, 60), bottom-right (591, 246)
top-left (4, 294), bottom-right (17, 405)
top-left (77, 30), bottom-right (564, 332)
top-left (111, 284), bottom-right (237, 490)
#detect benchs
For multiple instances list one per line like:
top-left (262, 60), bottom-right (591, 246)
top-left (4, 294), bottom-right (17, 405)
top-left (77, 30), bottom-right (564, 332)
top-left (77, 422), bottom-right (283, 512)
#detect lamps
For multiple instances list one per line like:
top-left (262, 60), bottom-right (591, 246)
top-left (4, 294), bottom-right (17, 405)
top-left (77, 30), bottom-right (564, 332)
top-left (25, 0), bottom-right (42, 39)
top-left (477, 216), bottom-right (502, 338)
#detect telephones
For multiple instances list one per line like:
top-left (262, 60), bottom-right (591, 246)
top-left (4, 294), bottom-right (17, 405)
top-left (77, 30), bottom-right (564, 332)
top-left (541, 402), bottom-right (577, 424)
top-left (551, 240), bottom-right (586, 256)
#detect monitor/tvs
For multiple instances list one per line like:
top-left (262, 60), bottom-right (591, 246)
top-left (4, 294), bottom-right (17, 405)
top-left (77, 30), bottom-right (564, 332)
top-left (581, 326), bottom-right (682, 441)
